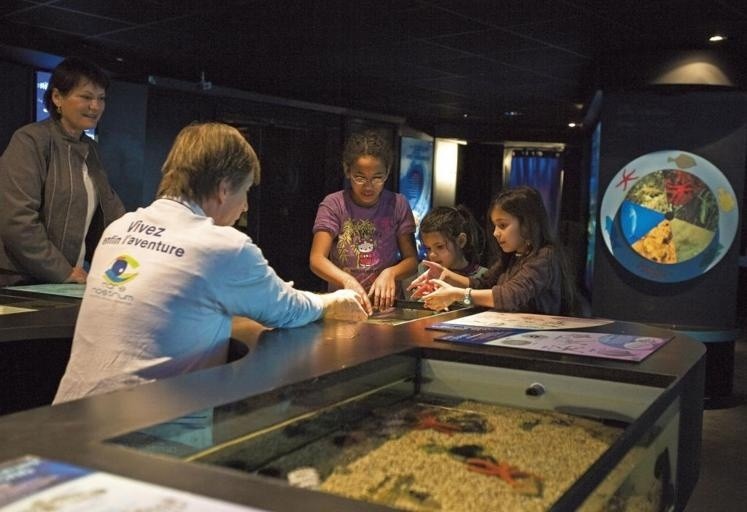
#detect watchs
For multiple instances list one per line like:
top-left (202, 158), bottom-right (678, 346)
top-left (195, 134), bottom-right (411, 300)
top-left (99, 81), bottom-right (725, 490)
top-left (463, 288), bottom-right (472, 305)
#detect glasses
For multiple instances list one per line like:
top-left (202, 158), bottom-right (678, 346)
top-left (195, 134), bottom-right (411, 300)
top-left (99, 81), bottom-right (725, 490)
top-left (350, 175), bottom-right (385, 184)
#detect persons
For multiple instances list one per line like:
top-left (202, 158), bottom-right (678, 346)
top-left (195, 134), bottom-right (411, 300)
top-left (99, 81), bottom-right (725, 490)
top-left (411, 185), bottom-right (563, 315)
top-left (309, 120), bottom-right (419, 316)
top-left (415, 204), bottom-right (490, 309)
top-left (0, 54), bottom-right (129, 289)
top-left (50, 121), bottom-right (369, 449)
